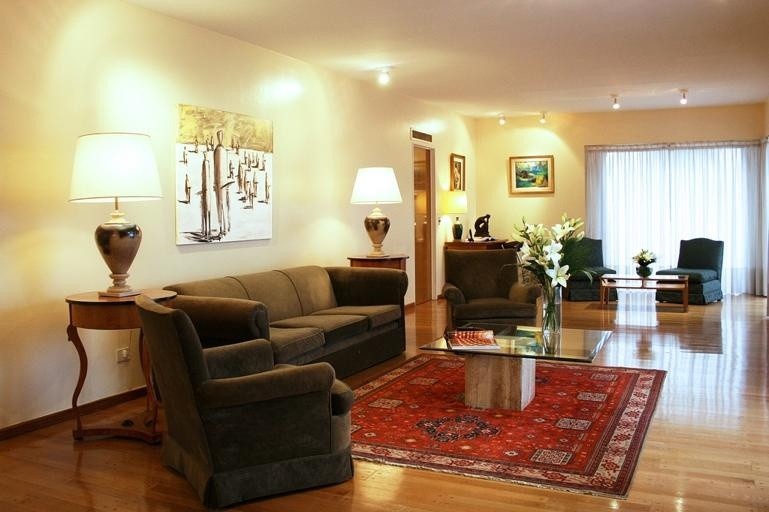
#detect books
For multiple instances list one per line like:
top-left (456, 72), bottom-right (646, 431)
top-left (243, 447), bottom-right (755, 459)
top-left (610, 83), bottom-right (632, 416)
top-left (444, 326), bottom-right (500, 351)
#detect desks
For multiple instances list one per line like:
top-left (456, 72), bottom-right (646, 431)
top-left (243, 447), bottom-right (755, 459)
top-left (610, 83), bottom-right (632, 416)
top-left (346, 255), bottom-right (410, 351)
top-left (65, 288), bottom-right (180, 447)
top-left (599, 273), bottom-right (690, 313)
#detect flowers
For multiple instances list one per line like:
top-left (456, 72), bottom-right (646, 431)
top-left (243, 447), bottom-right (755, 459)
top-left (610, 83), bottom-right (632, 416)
top-left (632, 248), bottom-right (656, 264)
top-left (511, 211), bottom-right (606, 333)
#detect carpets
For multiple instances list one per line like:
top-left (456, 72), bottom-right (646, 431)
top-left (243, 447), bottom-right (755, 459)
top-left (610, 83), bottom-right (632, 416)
top-left (346, 351), bottom-right (667, 499)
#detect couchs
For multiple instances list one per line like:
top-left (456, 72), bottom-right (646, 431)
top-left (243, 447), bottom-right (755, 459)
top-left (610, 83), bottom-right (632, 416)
top-left (154, 264), bottom-right (409, 381)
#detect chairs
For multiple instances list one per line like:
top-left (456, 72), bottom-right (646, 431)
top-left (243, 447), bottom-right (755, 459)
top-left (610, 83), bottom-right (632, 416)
top-left (655, 237), bottom-right (725, 305)
top-left (553, 236), bottom-right (618, 302)
top-left (132, 294), bottom-right (358, 508)
top-left (442, 248), bottom-right (543, 327)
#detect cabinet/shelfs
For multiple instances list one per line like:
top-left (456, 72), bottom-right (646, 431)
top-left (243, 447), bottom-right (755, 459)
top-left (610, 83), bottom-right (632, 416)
top-left (444, 240), bottom-right (508, 250)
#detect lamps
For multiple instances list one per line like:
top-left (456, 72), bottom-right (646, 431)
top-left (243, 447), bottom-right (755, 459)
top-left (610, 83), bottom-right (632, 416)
top-left (539, 110), bottom-right (548, 123)
top-left (439, 190), bottom-right (470, 240)
top-left (350, 167), bottom-right (403, 257)
top-left (611, 96), bottom-right (620, 109)
top-left (66, 130), bottom-right (163, 297)
top-left (680, 88), bottom-right (689, 104)
top-left (498, 112), bottom-right (505, 124)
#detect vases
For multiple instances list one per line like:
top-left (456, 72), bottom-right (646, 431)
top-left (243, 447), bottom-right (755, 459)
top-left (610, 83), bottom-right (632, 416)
top-left (542, 285), bottom-right (562, 334)
top-left (635, 266), bottom-right (653, 276)
top-left (638, 258), bottom-right (655, 267)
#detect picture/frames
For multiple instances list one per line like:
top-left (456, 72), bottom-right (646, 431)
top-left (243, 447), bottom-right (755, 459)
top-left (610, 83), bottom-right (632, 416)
top-left (508, 154), bottom-right (555, 194)
top-left (449, 152), bottom-right (466, 191)
